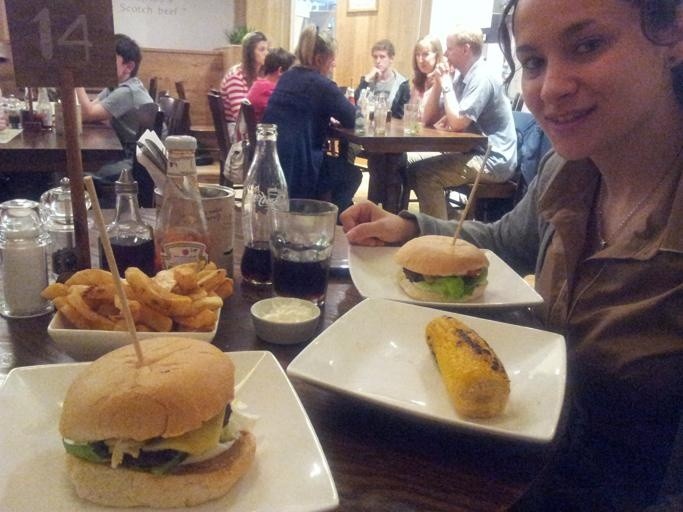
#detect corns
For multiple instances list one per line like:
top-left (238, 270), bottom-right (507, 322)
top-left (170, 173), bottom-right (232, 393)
top-left (426, 314), bottom-right (509, 418)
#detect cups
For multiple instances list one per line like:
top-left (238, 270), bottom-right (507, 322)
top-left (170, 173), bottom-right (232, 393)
top-left (271, 199), bottom-right (339, 306)
top-left (403, 103), bottom-right (419, 136)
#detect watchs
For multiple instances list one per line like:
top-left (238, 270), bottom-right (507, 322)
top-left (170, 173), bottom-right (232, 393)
top-left (442, 86), bottom-right (453, 94)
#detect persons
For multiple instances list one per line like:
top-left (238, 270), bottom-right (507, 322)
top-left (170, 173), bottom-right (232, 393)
top-left (235, 47), bottom-right (295, 141)
top-left (353, 38), bottom-right (411, 204)
top-left (337, 0), bottom-right (683, 512)
top-left (219, 31), bottom-right (268, 144)
top-left (387, 33), bottom-right (443, 214)
top-left (262, 22), bottom-right (362, 225)
top-left (407, 26), bottom-right (519, 222)
top-left (74, 32), bottom-right (169, 208)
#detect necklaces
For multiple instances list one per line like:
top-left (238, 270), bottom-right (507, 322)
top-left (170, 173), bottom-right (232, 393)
top-left (592, 153), bottom-right (681, 251)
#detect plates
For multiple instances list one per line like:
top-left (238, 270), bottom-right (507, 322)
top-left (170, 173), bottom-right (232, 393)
top-left (252, 296), bottom-right (320, 345)
top-left (345, 243), bottom-right (545, 310)
top-left (4, 349), bottom-right (342, 508)
top-left (286, 299), bottom-right (568, 446)
top-left (47, 295), bottom-right (221, 349)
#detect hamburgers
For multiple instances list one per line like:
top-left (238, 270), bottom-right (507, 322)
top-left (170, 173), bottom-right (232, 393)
top-left (395, 234), bottom-right (488, 303)
top-left (60, 335), bottom-right (255, 511)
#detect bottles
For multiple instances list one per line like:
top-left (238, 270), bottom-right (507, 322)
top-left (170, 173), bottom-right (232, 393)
top-left (38, 176), bottom-right (95, 282)
top-left (153, 179), bottom-right (236, 285)
top-left (7, 86), bottom-right (52, 131)
top-left (154, 133), bottom-right (209, 272)
top-left (243, 122), bottom-right (290, 287)
top-left (98, 166), bottom-right (158, 277)
top-left (0, 197), bottom-right (51, 322)
top-left (345, 86), bottom-right (390, 135)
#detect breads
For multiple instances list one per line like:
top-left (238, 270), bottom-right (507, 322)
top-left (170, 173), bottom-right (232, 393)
top-left (43, 259), bottom-right (232, 331)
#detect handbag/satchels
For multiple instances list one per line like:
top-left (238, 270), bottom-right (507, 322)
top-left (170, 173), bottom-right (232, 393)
top-left (222, 140), bottom-right (250, 184)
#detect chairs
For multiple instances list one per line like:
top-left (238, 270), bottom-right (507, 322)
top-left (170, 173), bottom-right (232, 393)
top-left (148, 78), bottom-right (158, 100)
top-left (240, 100), bottom-right (259, 165)
top-left (207, 92), bottom-right (244, 189)
top-left (446, 109), bottom-right (548, 217)
top-left (154, 95), bottom-right (190, 136)
top-left (175, 81), bottom-right (215, 185)
